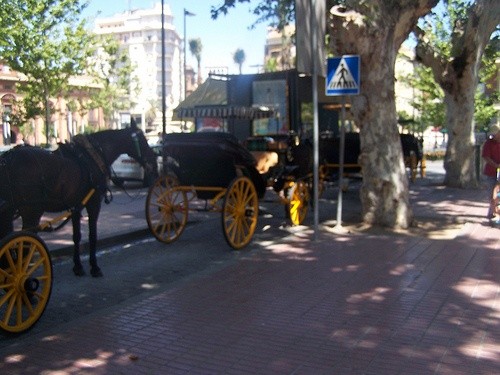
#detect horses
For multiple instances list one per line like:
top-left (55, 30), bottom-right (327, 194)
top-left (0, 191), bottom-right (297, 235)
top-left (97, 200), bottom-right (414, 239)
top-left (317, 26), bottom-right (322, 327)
top-left (0, 118), bottom-right (158, 292)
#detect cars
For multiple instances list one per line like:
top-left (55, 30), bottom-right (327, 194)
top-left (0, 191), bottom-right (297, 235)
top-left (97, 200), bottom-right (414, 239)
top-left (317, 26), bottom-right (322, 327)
top-left (111, 145), bottom-right (179, 189)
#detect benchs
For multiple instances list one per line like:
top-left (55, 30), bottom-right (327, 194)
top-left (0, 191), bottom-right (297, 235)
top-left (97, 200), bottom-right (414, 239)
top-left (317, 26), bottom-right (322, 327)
top-left (326, 132), bottom-right (361, 174)
top-left (162, 131), bottom-right (265, 199)
top-left (400, 134), bottom-right (418, 157)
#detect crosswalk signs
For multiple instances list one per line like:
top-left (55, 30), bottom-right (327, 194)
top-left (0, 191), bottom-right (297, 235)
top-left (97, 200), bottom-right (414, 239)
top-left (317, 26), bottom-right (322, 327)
top-left (325, 56), bottom-right (359, 96)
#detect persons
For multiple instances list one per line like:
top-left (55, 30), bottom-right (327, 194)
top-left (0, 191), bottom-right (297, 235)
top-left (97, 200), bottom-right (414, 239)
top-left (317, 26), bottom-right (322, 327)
top-left (482, 130), bottom-right (500, 218)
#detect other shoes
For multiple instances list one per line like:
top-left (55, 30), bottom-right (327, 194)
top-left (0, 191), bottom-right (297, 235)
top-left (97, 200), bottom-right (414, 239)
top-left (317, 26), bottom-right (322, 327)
top-left (488, 214), bottom-right (496, 218)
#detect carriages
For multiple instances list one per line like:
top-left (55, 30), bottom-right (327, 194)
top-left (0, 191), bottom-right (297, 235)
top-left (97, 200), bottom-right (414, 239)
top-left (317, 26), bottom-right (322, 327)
top-left (1, 116), bottom-right (158, 336)
top-left (397, 119), bottom-right (426, 182)
